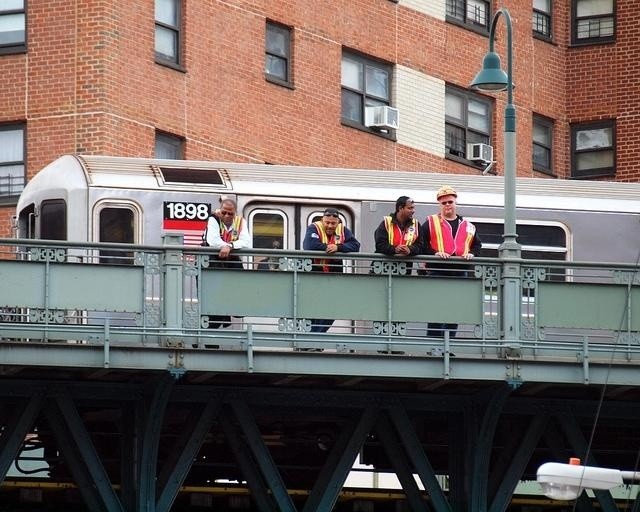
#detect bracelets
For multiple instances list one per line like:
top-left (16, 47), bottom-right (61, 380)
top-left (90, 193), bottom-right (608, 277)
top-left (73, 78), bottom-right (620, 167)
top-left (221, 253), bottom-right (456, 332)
top-left (227, 244), bottom-right (233, 248)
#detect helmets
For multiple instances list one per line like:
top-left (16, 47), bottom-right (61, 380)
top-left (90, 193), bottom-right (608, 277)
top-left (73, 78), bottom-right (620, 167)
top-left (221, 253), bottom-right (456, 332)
top-left (437, 185), bottom-right (457, 200)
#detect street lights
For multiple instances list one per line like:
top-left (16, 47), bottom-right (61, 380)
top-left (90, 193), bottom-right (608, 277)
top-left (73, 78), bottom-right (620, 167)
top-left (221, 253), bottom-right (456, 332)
top-left (472, 7), bottom-right (526, 361)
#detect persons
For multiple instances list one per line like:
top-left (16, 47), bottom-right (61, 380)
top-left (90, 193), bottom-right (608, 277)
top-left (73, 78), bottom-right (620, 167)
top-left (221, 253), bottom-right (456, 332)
top-left (369, 196), bottom-right (424, 354)
top-left (192, 200), bottom-right (250, 349)
top-left (300, 208), bottom-right (361, 351)
top-left (421, 185), bottom-right (482, 355)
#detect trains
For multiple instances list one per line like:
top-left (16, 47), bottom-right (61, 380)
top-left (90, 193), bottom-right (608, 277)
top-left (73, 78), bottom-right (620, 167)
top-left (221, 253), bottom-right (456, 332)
top-left (11, 150), bottom-right (640, 344)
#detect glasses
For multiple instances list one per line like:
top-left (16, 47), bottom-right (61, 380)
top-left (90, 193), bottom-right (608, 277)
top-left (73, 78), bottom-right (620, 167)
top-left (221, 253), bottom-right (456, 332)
top-left (324, 212), bottom-right (338, 217)
top-left (220, 209), bottom-right (234, 216)
top-left (441, 200), bottom-right (453, 205)
